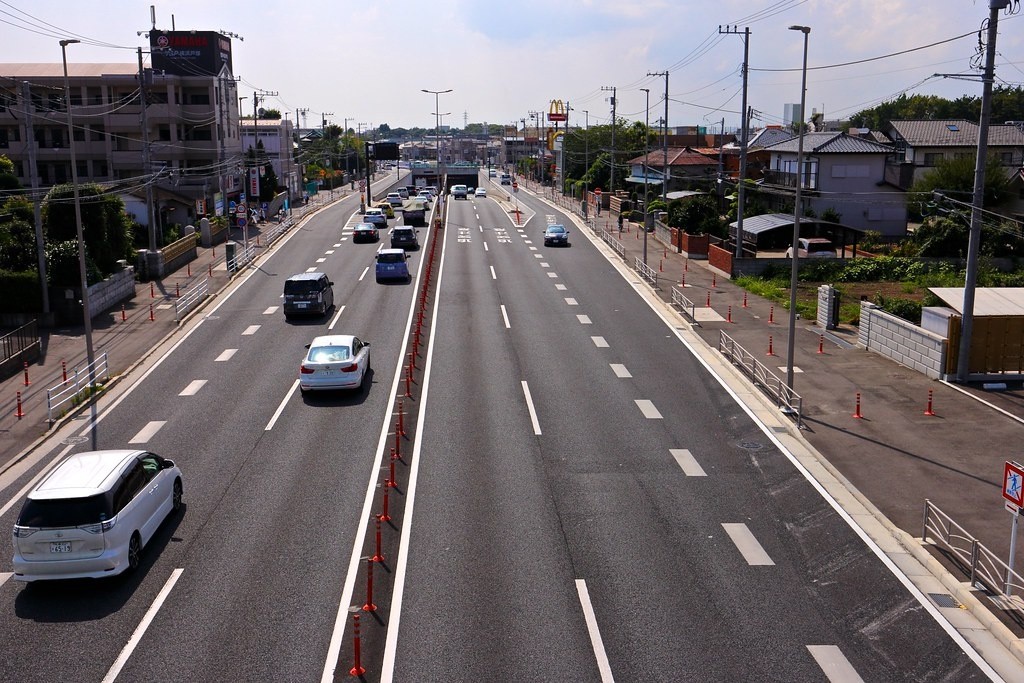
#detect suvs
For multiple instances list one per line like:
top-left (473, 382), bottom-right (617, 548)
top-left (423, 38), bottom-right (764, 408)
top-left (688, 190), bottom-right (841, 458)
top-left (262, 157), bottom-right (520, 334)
top-left (11, 448), bottom-right (185, 584)
top-left (375, 248), bottom-right (410, 282)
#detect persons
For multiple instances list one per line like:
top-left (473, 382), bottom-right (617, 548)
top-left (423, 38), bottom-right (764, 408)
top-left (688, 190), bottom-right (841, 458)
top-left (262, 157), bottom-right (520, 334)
top-left (596, 201), bottom-right (601, 214)
top-left (617, 215), bottom-right (623, 228)
top-left (260, 211), bottom-right (286, 225)
top-left (304, 194), bottom-right (310, 203)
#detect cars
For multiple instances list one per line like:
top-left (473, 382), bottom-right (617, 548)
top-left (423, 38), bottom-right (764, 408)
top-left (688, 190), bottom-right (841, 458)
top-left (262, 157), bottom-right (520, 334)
top-left (383, 164), bottom-right (392, 170)
top-left (353, 222), bottom-right (379, 242)
top-left (391, 226), bottom-right (419, 249)
top-left (785, 236), bottom-right (837, 258)
top-left (298, 334), bottom-right (371, 397)
top-left (376, 178), bottom-right (487, 219)
top-left (542, 225), bottom-right (570, 247)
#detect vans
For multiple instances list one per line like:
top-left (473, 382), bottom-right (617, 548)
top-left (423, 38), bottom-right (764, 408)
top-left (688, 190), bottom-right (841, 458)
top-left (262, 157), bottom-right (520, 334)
top-left (283, 273), bottom-right (335, 316)
top-left (490, 169), bottom-right (496, 178)
top-left (500, 174), bottom-right (512, 185)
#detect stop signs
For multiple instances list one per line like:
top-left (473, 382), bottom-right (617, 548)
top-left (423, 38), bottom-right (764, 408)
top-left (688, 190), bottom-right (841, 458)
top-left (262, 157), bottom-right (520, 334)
top-left (594, 188), bottom-right (601, 195)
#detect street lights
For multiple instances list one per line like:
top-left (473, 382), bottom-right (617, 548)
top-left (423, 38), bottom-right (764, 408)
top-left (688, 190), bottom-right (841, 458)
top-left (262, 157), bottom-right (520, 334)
top-left (640, 88), bottom-right (649, 278)
top-left (784, 23), bottom-right (812, 410)
top-left (239, 96), bottom-right (248, 248)
top-left (583, 110), bottom-right (588, 203)
top-left (285, 112), bottom-right (292, 215)
top-left (58, 38), bottom-right (97, 387)
top-left (421, 90), bottom-right (453, 216)
top-left (432, 112), bottom-right (451, 189)
